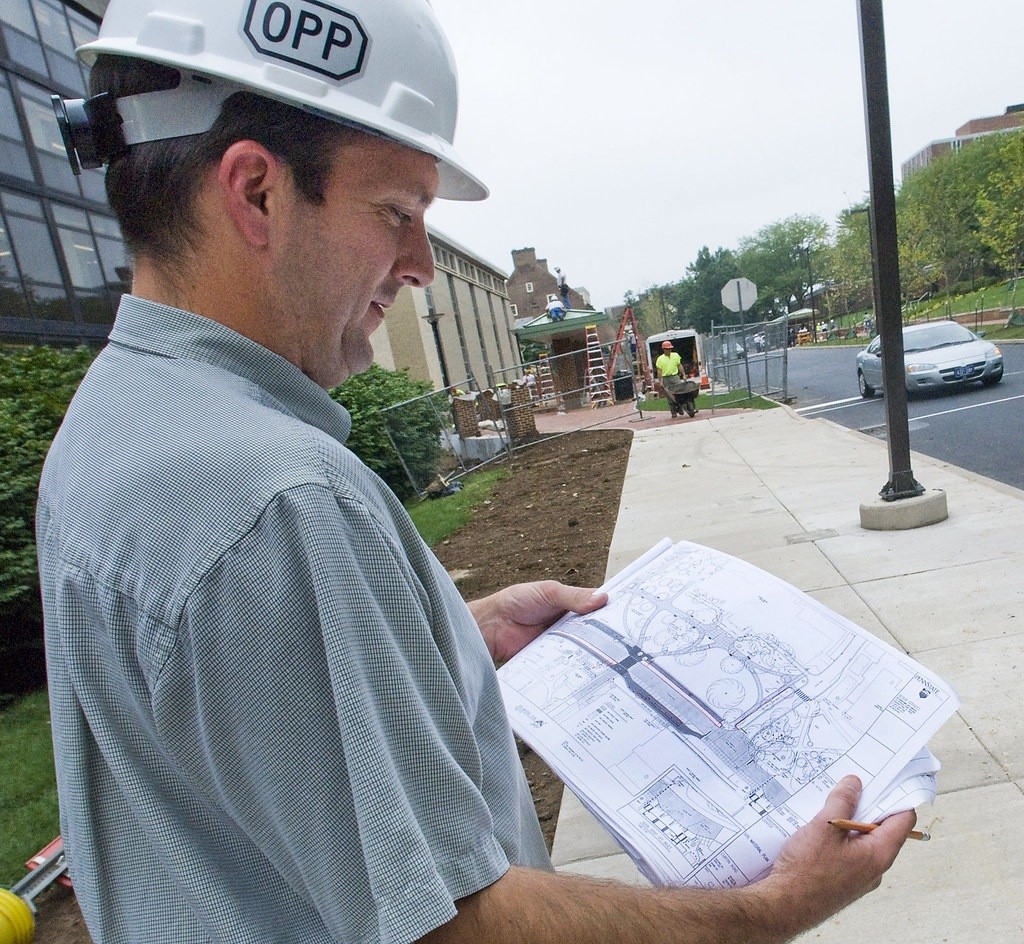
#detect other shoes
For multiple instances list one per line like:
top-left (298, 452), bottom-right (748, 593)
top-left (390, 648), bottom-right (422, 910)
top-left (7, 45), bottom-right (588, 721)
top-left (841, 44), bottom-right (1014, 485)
top-left (679, 409), bottom-right (684, 415)
top-left (559, 316), bottom-right (564, 321)
top-left (672, 413), bottom-right (677, 417)
top-left (552, 316), bottom-right (558, 322)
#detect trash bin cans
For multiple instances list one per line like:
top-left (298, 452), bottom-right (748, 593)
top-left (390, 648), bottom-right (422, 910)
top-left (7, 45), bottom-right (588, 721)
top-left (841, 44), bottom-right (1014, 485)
top-left (613, 372), bottom-right (634, 401)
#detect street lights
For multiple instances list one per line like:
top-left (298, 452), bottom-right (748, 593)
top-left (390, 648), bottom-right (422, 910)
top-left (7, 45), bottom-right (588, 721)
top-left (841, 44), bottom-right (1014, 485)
top-left (850, 205), bottom-right (879, 336)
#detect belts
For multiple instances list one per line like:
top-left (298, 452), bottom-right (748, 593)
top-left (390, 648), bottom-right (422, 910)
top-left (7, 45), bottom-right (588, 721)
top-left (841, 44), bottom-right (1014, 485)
top-left (663, 374), bottom-right (679, 377)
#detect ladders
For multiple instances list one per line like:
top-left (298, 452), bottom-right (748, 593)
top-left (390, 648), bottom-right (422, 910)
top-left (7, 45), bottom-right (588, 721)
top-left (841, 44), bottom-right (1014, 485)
top-left (585, 324), bottom-right (615, 410)
top-left (598, 306), bottom-right (652, 408)
top-left (538, 353), bottom-right (558, 407)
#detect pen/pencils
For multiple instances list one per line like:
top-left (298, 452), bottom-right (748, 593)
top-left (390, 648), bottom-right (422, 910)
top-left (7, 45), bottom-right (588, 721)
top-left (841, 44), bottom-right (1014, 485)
top-left (828, 819), bottom-right (931, 842)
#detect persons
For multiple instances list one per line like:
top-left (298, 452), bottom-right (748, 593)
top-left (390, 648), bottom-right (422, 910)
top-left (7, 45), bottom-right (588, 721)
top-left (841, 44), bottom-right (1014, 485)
top-left (544, 295), bottom-right (568, 323)
top-left (862, 312), bottom-right (872, 331)
top-left (622, 320), bottom-right (639, 362)
top-left (753, 333), bottom-right (762, 351)
top-left (554, 265), bottom-right (574, 309)
top-left (33, 1), bottom-right (920, 943)
top-left (655, 341), bottom-right (688, 418)
top-left (815, 319), bottom-right (836, 332)
top-left (522, 368), bottom-right (537, 407)
top-left (448, 385), bottom-right (465, 434)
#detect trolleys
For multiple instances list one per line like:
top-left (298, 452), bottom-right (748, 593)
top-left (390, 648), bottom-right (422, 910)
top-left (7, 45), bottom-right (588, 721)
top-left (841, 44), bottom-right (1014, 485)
top-left (661, 378), bottom-right (699, 417)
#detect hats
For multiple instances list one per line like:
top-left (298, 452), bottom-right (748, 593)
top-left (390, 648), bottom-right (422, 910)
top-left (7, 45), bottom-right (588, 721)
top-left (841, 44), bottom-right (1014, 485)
top-left (554, 266), bottom-right (560, 271)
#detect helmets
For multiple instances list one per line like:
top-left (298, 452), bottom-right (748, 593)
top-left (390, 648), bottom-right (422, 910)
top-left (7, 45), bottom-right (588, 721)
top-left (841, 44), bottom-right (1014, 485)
top-left (51, 0), bottom-right (490, 201)
top-left (551, 296), bottom-right (558, 301)
top-left (662, 341), bottom-right (673, 348)
top-left (821, 322), bottom-right (824, 324)
top-left (817, 323), bottom-right (820, 325)
top-left (755, 334), bottom-right (758, 336)
top-left (831, 319), bottom-right (834, 322)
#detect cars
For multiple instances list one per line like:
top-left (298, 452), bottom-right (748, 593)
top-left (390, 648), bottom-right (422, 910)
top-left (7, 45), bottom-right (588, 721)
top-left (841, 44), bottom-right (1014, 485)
top-left (718, 343), bottom-right (746, 360)
top-left (856, 320), bottom-right (1004, 398)
top-left (744, 331), bottom-right (776, 352)
